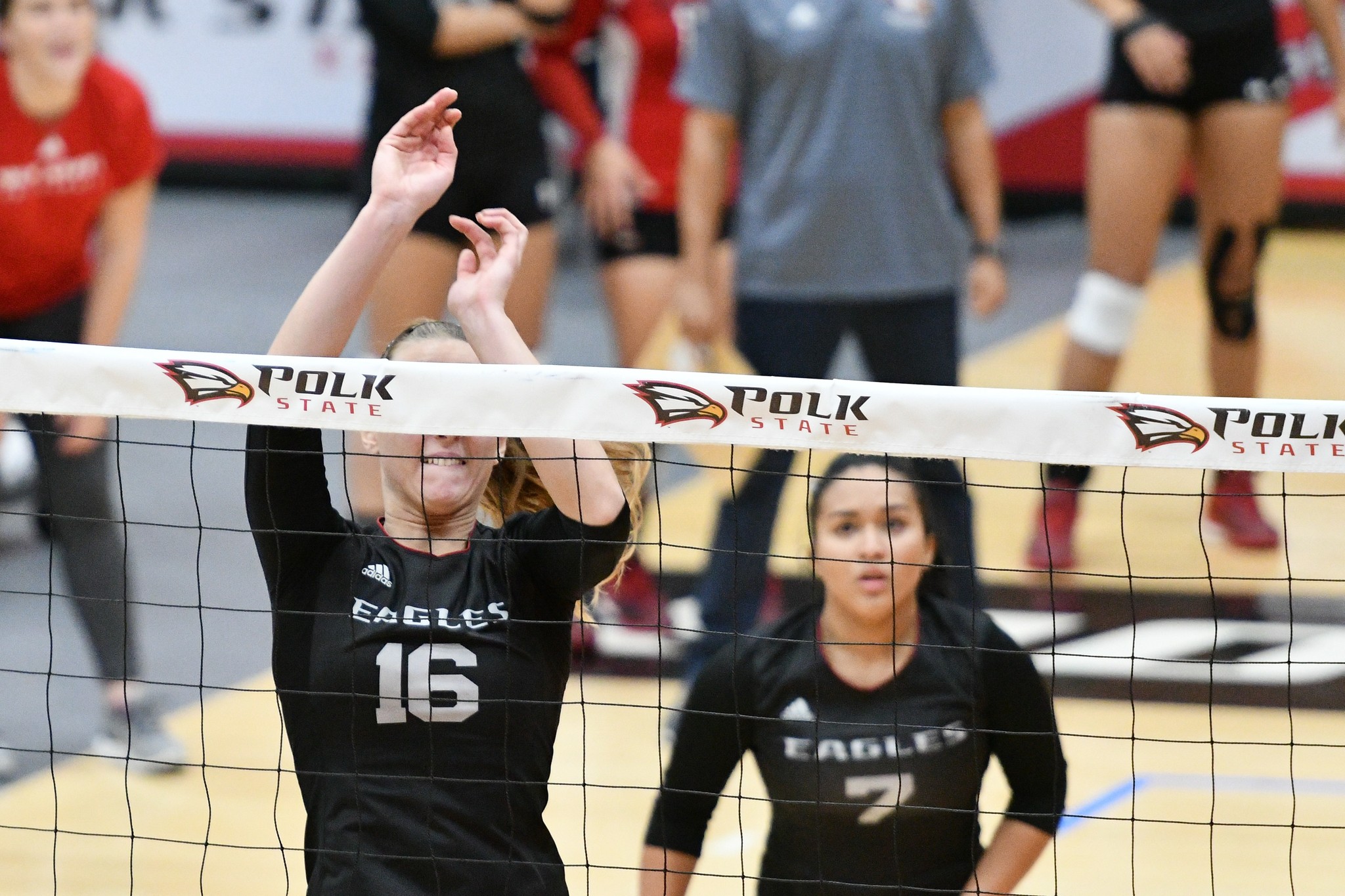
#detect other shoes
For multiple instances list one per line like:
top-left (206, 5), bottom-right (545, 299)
top-left (1025, 479), bottom-right (1079, 571)
top-left (0, 746), bottom-right (14, 779)
top-left (1202, 481), bottom-right (1280, 547)
top-left (92, 705), bottom-right (190, 776)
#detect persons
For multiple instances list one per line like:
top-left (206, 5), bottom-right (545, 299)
top-left (637, 452), bottom-right (1070, 895)
top-left (243, 85), bottom-right (634, 896)
top-left (356, 1), bottom-right (1012, 735)
top-left (1024, 0), bottom-right (1345, 576)
top-left (0, 0), bottom-right (188, 773)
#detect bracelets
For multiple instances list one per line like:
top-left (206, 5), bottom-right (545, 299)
top-left (968, 240), bottom-right (1009, 260)
top-left (1113, 15), bottom-right (1160, 50)
